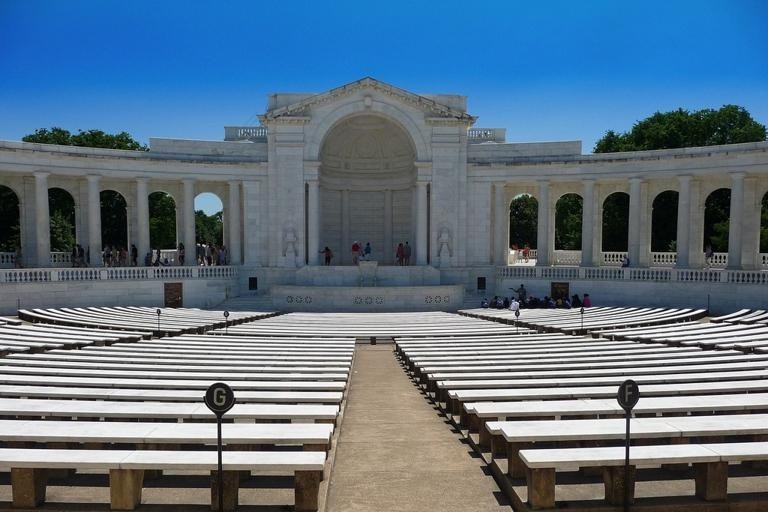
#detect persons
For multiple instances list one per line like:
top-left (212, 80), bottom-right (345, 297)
top-left (703, 237), bottom-right (715, 268)
top-left (516, 245), bottom-right (530, 262)
top-left (321, 248), bottom-right (335, 262)
top-left (350, 240), bottom-right (370, 264)
top-left (14, 245), bottom-right (23, 265)
top-left (71, 239), bottom-right (228, 266)
top-left (480, 283), bottom-right (590, 310)
top-left (620, 255), bottom-right (629, 267)
top-left (397, 242), bottom-right (410, 266)
